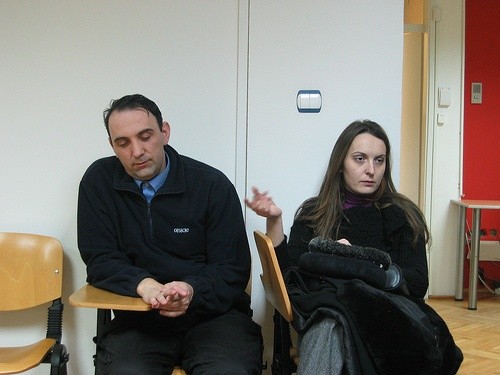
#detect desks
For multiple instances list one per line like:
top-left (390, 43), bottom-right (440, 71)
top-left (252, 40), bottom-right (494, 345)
top-left (448, 198), bottom-right (500, 311)
top-left (68, 280), bottom-right (155, 312)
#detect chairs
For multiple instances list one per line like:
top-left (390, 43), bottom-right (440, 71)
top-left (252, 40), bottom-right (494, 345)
top-left (252, 230), bottom-right (304, 375)
top-left (0, 231), bottom-right (69, 375)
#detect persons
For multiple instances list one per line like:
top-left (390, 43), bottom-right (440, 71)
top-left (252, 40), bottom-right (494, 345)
top-left (77, 93), bottom-right (266, 375)
top-left (244, 118), bottom-right (430, 375)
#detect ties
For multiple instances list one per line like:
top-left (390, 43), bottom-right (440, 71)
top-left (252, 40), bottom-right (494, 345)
top-left (142, 182), bottom-right (155, 204)
